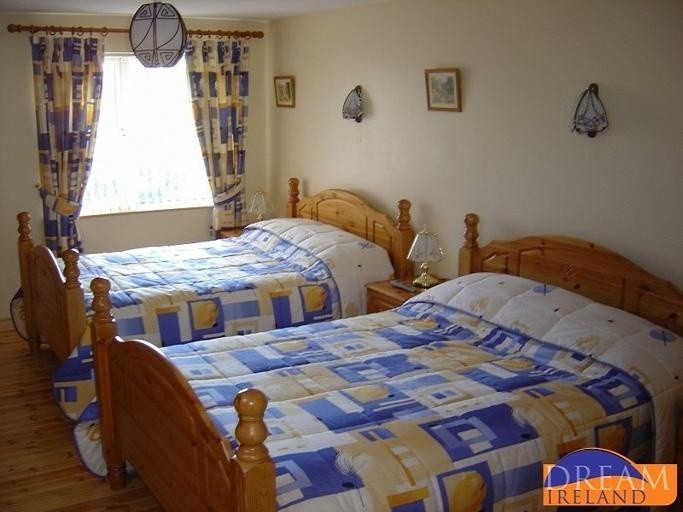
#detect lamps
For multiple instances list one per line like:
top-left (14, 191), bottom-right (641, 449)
top-left (342, 85), bottom-right (362, 123)
top-left (249, 189), bottom-right (276, 222)
top-left (407, 223), bottom-right (448, 289)
top-left (568, 83), bottom-right (609, 137)
top-left (128, 3), bottom-right (187, 68)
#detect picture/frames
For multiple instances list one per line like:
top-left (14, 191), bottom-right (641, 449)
top-left (273, 76), bottom-right (295, 107)
top-left (424, 67), bottom-right (462, 111)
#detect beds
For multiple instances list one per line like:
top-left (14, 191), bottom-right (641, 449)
top-left (89, 214), bottom-right (682, 511)
top-left (17, 178), bottom-right (412, 365)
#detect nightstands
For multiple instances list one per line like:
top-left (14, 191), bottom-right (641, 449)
top-left (216, 226), bottom-right (245, 239)
top-left (363, 274), bottom-right (448, 314)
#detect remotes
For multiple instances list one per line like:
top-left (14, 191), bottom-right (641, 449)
top-left (390, 278), bottom-right (417, 293)
